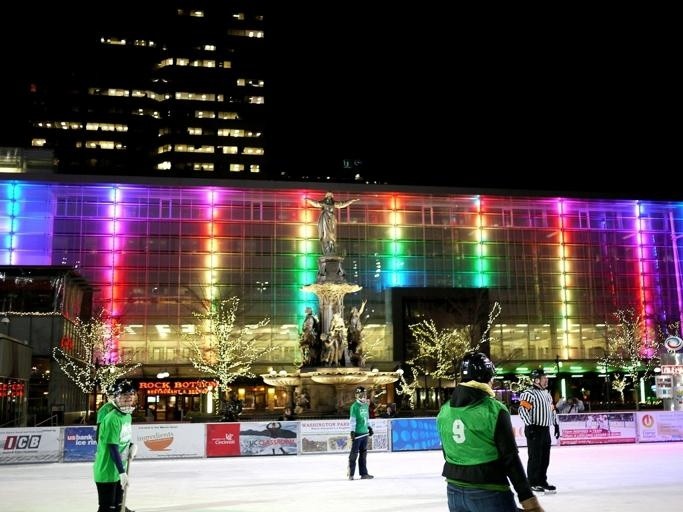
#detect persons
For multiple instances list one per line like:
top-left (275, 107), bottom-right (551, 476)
top-left (90, 380), bottom-right (140, 511)
top-left (265, 422), bottom-right (288, 454)
top-left (434, 353), bottom-right (545, 511)
top-left (556, 395), bottom-right (586, 413)
top-left (345, 386), bottom-right (375, 479)
top-left (583, 415), bottom-right (610, 434)
top-left (302, 193), bottom-right (359, 254)
top-left (517, 369), bottom-right (561, 493)
top-left (96, 381), bottom-right (124, 442)
top-left (297, 298), bottom-right (366, 368)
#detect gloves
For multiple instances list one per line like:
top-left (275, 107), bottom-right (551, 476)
top-left (350, 431), bottom-right (356, 441)
top-left (129, 443), bottom-right (138, 461)
top-left (367, 426), bottom-right (374, 436)
top-left (119, 472), bottom-right (130, 490)
top-left (553, 424), bottom-right (560, 439)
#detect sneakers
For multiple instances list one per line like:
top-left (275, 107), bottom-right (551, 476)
top-left (530, 484), bottom-right (544, 492)
top-left (540, 483), bottom-right (555, 490)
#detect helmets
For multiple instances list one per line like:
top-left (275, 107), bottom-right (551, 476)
top-left (460, 350), bottom-right (495, 383)
top-left (528, 368), bottom-right (545, 382)
top-left (113, 381), bottom-right (137, 414)
top-left (106, 384), bottom-right (120, 400)
top-left (354, 386), bottom-right (367, 403)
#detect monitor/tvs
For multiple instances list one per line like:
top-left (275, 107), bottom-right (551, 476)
top-left (147, 395), bottom-right (159, 404)
